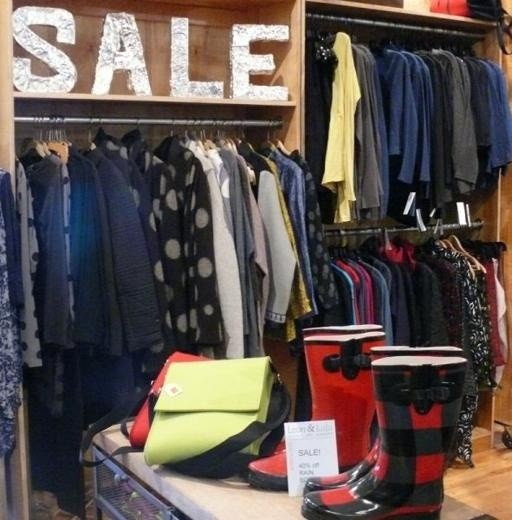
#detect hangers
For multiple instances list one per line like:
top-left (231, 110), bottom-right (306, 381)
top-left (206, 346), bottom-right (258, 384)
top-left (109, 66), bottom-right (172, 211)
top-left (23, 109), bottom-right (296, 160)
top-left (325, 220), bottom-right (506, 272)
top-left (307, 9), bottom-right (484, 58)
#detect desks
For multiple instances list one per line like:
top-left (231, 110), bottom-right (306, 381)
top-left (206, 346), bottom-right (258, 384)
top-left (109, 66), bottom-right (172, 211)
top-left (92, 419), bottom-right (504, 520)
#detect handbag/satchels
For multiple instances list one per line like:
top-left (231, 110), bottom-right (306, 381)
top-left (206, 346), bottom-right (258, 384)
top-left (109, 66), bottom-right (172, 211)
top-left (144, 355), bottom-right (284, 468)
top-left (130, 350), bottom-right (216, 449)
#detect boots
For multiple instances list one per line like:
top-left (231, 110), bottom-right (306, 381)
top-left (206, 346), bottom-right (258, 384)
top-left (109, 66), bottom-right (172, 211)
top-left (301, 356), bottom-right (467, 520)
top-left (270, 323), bottom-right (384, 457)
top-left (247, 331), bottom-right (387, 491)
top-left (304, 346), bottom-right (464, 499)
